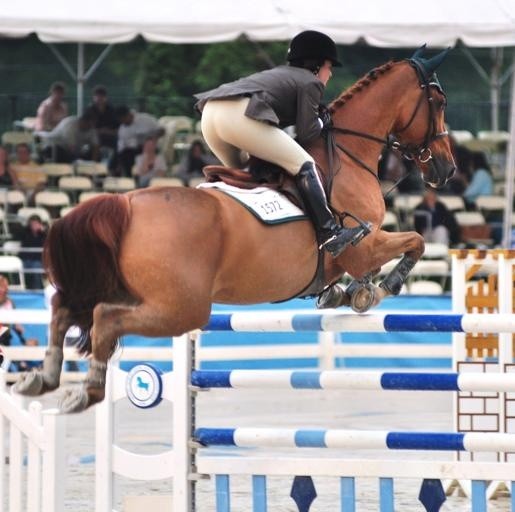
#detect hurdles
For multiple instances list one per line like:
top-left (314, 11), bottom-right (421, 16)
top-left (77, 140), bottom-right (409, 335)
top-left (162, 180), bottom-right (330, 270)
top-left (0, 310), bottom-right (515, 512)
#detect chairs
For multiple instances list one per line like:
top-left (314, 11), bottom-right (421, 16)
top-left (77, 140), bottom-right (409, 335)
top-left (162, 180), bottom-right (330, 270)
top-left (0, 111), bottom-right (203, 299)
top-left (379, 131), bottom-right (513, 295)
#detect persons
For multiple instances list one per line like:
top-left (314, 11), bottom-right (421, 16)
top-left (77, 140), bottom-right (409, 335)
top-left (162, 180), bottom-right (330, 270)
top-left (380, 130), bottom-right (514, 289)
top-left (191, 31), bottom-right (373, 258)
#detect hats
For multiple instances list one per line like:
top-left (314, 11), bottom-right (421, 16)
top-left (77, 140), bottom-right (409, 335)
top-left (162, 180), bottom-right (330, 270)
top-left (286, 30), bottom-right (345, 68)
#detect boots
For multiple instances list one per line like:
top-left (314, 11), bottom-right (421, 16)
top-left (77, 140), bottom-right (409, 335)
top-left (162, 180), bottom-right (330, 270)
top-left (293, 161), bottom-right (373, 259)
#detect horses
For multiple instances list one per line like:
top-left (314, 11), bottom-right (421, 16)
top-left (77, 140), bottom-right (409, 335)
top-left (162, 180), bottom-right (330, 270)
top-left (14, 40), bottom-right (460, 420)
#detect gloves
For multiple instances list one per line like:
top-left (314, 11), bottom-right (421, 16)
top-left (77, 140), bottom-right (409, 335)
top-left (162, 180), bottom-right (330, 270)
top-left (318, 104), bottom-right (333, 131)
